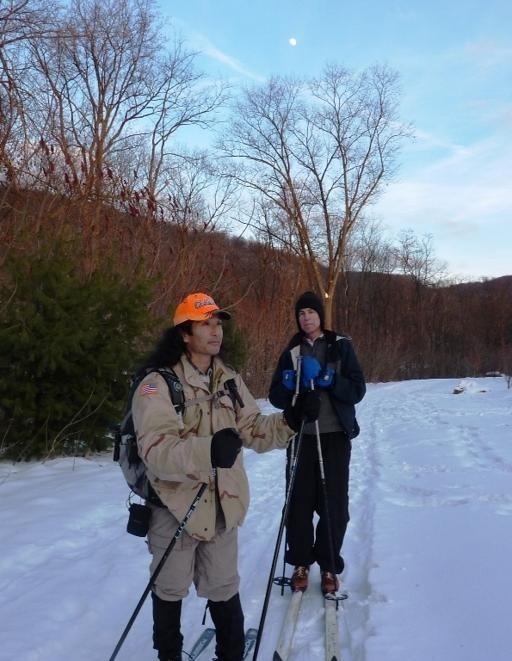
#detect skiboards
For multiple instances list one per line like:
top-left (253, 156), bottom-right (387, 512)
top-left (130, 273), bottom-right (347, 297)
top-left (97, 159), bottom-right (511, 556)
top-left (179, 628), bottom-right (258, 661)
top-left (271, 590), bottom-right (342, 660)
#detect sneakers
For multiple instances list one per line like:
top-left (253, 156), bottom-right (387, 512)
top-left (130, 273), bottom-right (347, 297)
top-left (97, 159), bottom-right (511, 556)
top-left (290, 566), bottom-right (309, 592)
top-left (320, 567), bottom-right (339, 594)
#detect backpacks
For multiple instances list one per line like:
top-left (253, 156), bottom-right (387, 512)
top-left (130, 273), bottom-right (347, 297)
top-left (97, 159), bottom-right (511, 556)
top-left (113, 364), bottom-right (245, 509)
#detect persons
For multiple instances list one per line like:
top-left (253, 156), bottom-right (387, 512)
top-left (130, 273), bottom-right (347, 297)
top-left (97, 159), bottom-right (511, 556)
top-left (270, 291), bottom-right (367, 593)
top-left (131, 293), bottom-right (321, 661)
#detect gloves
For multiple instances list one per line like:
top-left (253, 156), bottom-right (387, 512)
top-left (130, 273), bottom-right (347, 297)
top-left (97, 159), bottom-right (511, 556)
top-left (301, 356), bottom-right (335, 389)
top-left (284, 384), bottom-right (322, 434)
top-left (281, 370), bottom-right (310, 391)
top-left (211, 428), bottom-right (243, 469)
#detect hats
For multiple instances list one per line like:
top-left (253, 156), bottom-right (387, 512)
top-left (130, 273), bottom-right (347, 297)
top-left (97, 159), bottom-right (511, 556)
top-left (295, 291), bottom-right (324, 333)
top-left (173, 293), bottom-right (231, 327)
